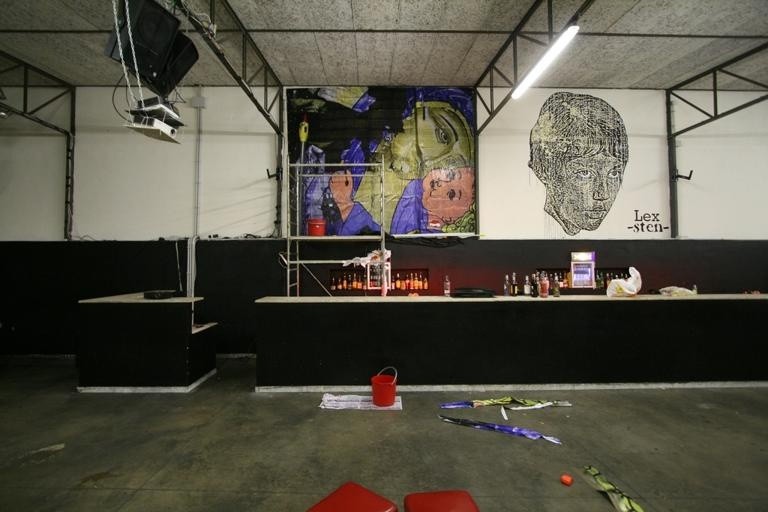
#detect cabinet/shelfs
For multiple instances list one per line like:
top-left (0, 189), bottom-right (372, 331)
top-left (533, 266), bottom-right (631, 295)
top-left (327, 267), bottom-right (430, 292)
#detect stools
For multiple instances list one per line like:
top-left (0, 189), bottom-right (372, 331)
top-left (307, 480), bottom-right (481, 512)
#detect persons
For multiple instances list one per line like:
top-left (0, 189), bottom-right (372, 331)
top-left (528, 92), bottom-right (630, 237)
top-left (286, 86), bottom-right (477, 183)
top-left (303, 165), bottom-right (476, 234)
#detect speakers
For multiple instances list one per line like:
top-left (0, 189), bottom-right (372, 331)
top-left (103, 1), bottom-right (181, 78)
top-left (138, 30), bottom-right (200, 98)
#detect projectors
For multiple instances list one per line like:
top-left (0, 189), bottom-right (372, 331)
top-left (143, 290), bottom-right (176, 299)
top-left (132, 115), bottom-right (178, 139)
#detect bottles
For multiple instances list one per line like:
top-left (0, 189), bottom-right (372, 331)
top-left (443, 274), bottom-right (451, 296)
top-left (503, 269), bottom-right (628, 297)
top-left (330, 266), bottom-right (428, 291)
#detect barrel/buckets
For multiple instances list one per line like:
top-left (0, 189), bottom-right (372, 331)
top-left (371, 367), bottom-right (398, 406)
